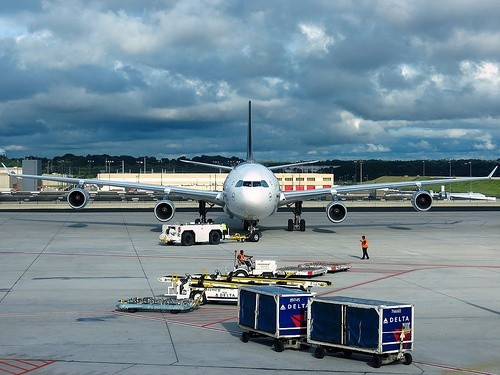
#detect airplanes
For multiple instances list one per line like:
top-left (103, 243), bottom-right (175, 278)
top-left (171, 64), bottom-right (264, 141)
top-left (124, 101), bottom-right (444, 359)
top-left (9, 99), bottom-right (500, 243)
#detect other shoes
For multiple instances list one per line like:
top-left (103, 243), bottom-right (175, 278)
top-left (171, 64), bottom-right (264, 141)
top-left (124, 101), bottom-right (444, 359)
top-left (361, 257), bottom-right (364, 259)
top-left (367, 257), bottom-right (369, 259)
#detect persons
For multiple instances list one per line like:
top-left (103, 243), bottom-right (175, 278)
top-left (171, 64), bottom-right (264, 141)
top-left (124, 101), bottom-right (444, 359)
top-left (237, 249), bottom-right (253, 271)
top-left (359, 236), bottom-right (370, 259)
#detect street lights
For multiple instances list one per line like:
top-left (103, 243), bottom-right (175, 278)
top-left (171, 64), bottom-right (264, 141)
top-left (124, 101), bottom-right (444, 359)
top-left (48, 161), bottom-right (50, 176)
top-left (448, 162), bottom-right (452, 179)
top-left (359, 161), bottom-right (363, 184)
top-left (105, 161), bottom-right (114, 191)
top-left (469, 162), bottom-right (472, 177)
